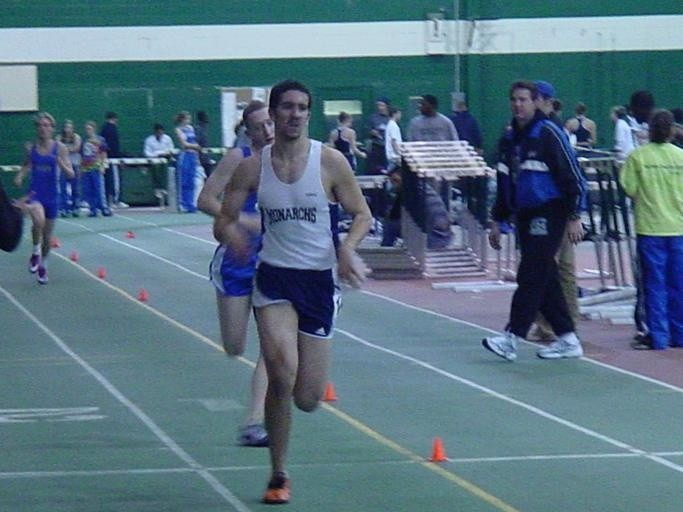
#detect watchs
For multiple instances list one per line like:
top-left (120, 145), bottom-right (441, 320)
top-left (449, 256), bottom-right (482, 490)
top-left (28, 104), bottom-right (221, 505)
top-left (569, 214), bottom-right (581, 221)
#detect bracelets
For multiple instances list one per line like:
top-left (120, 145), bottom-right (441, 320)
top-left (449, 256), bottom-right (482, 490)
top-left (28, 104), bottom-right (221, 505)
top-left (344, 237), bottom-right (361, 250)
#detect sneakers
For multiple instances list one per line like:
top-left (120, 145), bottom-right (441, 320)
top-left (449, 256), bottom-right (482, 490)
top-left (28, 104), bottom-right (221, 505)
top-left (36, 265), bottom-right (49, 284)
top-left (88, 212), bottom-right (97, 217)
top-left (263, 470), bottom-right (291, 503)
top-left (631, 335), bottom-right (653, 350)
top-left (116, 201), bottom-right (129, 209)
top-left (239, 422), bottom-right (269, 446)
top-left (28, 253), bottom-right (41, 273)
top-left (101, 209), bottom-right (111, 216)
top-left (60, 212), bottom-right (66, 217)
top-left (481, 334), bottom-right (518, 361)
top-left (71, 211), bottom-right (79, 217)
top-left (536, 338), bottom-right (584, 359)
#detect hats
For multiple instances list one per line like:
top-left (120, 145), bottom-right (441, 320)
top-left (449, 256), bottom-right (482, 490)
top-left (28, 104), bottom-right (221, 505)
top-left (533, 80), bottom-right (555, 97)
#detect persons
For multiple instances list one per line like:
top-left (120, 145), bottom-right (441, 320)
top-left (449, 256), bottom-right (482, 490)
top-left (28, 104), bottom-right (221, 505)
top-left (55, 118), bottom-right (81, 218)
top-left (328, 112), bottom-right (367, 174)
top-left (631, 123), bottom-right (683, 342)
top-left (99, 111), bottom-right (130, 209)
top-left (406, 96), bottom-right (459, 141)
top-left (0, 182), bottom-right (37, 252)
top-left (610, 105), bottom-right (632, 209)
top-left (385, 107), bottom-right (403, 173)
top-left (78, 121), bottom-right (112, 217)
top-left (562, 117), bottom-right (580, 148)
top-left (549, 98), bottom-right (563, 130)
top-left (520, 78), bottom-right (581, 343)
top-left (444, 99), bottom-right (483, 154)
top-left (143, 124), bottom-right (175, 199)
top-left (615, 90), bottom-right (654, 169)
top-left (172, 111), bottom-right (208, 213)
top-left (574, 103), bottom-right (596, 148)
top-left (620, 108), bottom-right (683, 349)
top-left (14, 112), bottom-right (75, 285)
top-left (482, 81), bottom-right (588, 363)
top-left (193, 112), bottom-right (215, 178)
top-left (233, 121), bottom-right (251, 146)
top-left (379, 167), bottom-right (452, 249)
top-left (366, 96), bottom-right (390, 175)
top-left (213, 80), bottom-right (373, 504)
top-left (197, 99), bottom-right (275, 447)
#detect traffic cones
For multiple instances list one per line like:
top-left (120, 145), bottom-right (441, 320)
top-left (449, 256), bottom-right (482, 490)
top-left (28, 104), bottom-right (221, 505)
top-left (98, 267), bottom-right (106, 278)
top-left (138, 286), bottom-right (147, 300)
top-left (428, 436), bottom-right (448, 461)
top-left (71, 252), bottom-right (77, 260)
top-left (321, 382), bottom-right (338, 401)
